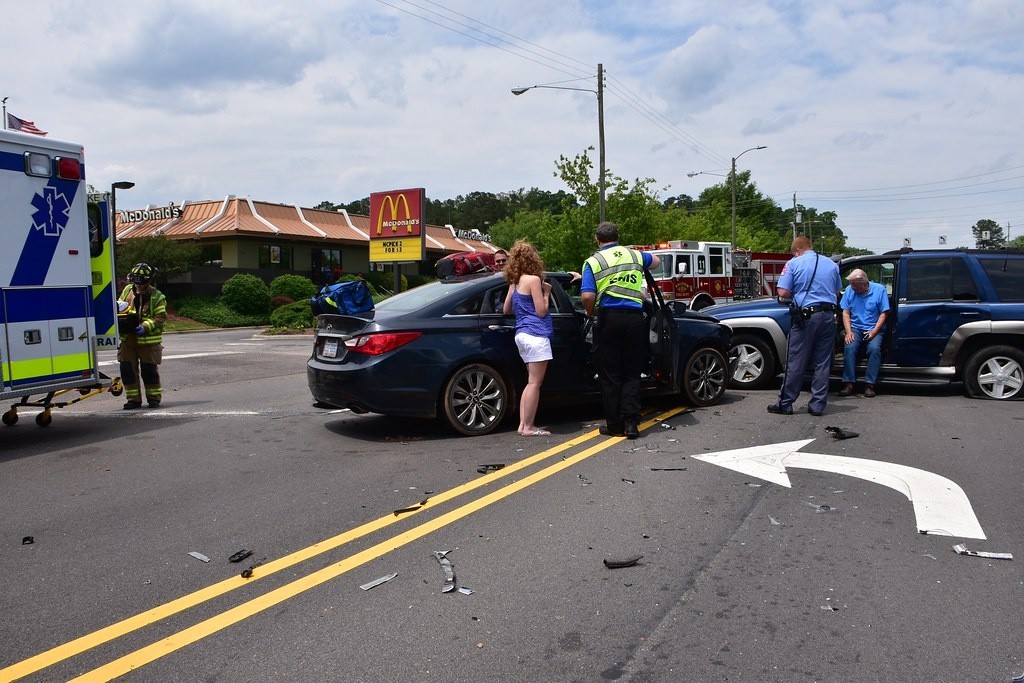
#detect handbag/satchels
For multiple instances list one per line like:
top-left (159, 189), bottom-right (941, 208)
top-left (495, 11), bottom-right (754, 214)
top-left (435, 251), bottom-right (495, 279)
top-left (311, 280), bottom-right (374, 316)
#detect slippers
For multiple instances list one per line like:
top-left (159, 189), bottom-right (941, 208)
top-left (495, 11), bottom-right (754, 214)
top-left (516, 426), bottom-right (551, 436)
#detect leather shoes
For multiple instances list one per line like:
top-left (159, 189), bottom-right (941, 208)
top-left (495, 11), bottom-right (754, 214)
top-left (840, 383), bottom-right (854, 396)
top-left (865, 384), bottom-right (875, 397)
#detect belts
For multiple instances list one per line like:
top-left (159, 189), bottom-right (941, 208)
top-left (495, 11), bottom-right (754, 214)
top-left (605, 308), bottom-right (641, 313)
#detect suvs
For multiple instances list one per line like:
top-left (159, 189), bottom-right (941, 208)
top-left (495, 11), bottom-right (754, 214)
top-left (697, 247), bottom-right (1024, 402)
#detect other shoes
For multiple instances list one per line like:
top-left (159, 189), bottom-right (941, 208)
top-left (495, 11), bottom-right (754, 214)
top-left (624, 427), bottom-right (639, 438)
top-left (600, 426), bottom-right (623, 436)
top-left (149, 401), bottom-right (158, 407)
top-left (807, 407), bottom-right (822, 416)
top-left (767, 405), bottom-right (793, 415)
top-left (124, 402), bottom-right (141, 409)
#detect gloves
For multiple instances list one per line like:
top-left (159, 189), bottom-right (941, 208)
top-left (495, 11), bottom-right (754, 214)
top-left (136, 325), bottom-right (145, 336)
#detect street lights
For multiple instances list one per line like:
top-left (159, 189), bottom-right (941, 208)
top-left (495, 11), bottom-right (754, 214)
top-left (686, 145), bottom-right (768, 246)
top-left (509, 63), bottom-right (606, 226)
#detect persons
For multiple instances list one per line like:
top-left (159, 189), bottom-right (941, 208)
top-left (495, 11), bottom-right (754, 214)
top-left (767, 236), bottom-right (843, 417)
top-left (116, 263), bottom-right (167, 408)
top-left (566, 271), bottom-right (582, 283)
top-left (503, 239), bottom-right (551, 437)
top-left (579, 221), bottom-right (661, 439)
top-left (840, 269), bottom-right (890, 397)
top-left (480, 249), bottom-right (508, 312)
top-left (322, 263), bottom-right (367, 283)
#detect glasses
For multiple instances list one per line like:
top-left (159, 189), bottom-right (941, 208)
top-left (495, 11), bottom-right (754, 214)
top-left (496, 259), bottom-right (506, 263)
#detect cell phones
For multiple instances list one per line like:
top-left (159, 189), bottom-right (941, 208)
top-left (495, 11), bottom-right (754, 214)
top-left (864, 333), bottom-right (869, 339)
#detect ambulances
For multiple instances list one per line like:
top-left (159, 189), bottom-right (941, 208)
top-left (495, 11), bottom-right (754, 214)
top-left (0, 128), bottom-right (121, 405)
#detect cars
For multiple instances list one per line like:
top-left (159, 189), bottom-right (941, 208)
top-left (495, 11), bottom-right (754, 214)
top-left (308, 268), bottom-right (735, 438)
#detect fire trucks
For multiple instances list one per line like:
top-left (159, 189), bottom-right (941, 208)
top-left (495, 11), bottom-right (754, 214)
top-left (629, 239), bottom-right (795, 309)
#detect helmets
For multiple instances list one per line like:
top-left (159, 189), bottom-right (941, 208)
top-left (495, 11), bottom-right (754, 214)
top-left (127, 262), bottom-right (158, 283)
